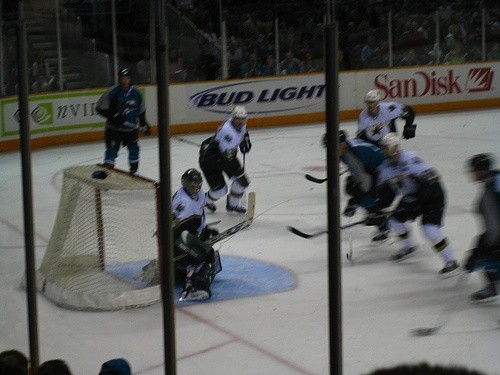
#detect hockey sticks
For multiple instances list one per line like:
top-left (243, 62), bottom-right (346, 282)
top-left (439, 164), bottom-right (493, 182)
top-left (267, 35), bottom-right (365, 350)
top-left (124, 121), bottom-right (199, 148)
top-left (305, 134), bottom-right (405, 184)
top-left (285, 207), bottom-right (406, 239)
top-left (242, 149), bottom-right (246, 171)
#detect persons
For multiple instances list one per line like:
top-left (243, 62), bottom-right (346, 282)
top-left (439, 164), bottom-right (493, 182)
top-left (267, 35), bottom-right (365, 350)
top-left (199, 106), bottom-right (252, 217)
top-left (95, 68), bottom-right (150, 175)
top-left (0, 350), bottom-right (27, 375)
top-left (464, 153), bottom-right (500, 306)
top-left (0, 48), bottom-right (73, 97)
top-left (321, 130), bottom-right (395, 246)
top-left (98, 358), bottom-right (131, 375)
top-left (38, 359), bottom-right (71, 375)
top-left (136, 0), bottom-right (500, 84)
top-left (363, 132), bottom-right (463, 279)
top-left (356, 89), bottom-right (416, 147)
top-left (171, 169), bottom-right (222, 302)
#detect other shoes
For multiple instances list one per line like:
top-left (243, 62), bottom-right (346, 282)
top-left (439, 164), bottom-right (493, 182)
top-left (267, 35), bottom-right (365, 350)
top-left (226, 194), bottom-right (247, 216)
top-left (439, 260), bottom-right (460, 279)
top-left (181, 284), bottom-right (211, 302)
top-left (370, 222), bottom-right (395, 243)
top-left (205, 192), bottom-right (217, 213)
top-left (388, 241), bottom-right (421, 264)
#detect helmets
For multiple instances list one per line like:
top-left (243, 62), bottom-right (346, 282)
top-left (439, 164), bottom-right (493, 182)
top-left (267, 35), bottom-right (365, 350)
top-left (119, 68), bottom-right (130, 78)
top-left (379, 133), bottom-right (403, 158)
top-left (230, 105), bottom-right (248, 120)
top-left (321, 129), bottom-right (347, 147)
top-left (181, 168), bottom-right (203, 186)
top-left (465, 153), bottom-right (494, 184)
top-left (363, 89), bottom-right (384, 106)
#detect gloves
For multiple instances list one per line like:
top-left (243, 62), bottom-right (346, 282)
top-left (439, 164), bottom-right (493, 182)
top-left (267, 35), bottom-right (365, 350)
top-left (139, 124), bottom-right (152, 137)
top-left (240, 134), bottom-right (252, 153)
top-left (402, 124), bottom-right (417, 139)
top-left (238, 169), bottom-right (250, 186)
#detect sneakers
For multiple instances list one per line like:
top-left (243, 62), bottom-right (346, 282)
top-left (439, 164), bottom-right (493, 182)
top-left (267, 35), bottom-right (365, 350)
top-left (471, 286), bottom-right (498, 304)
